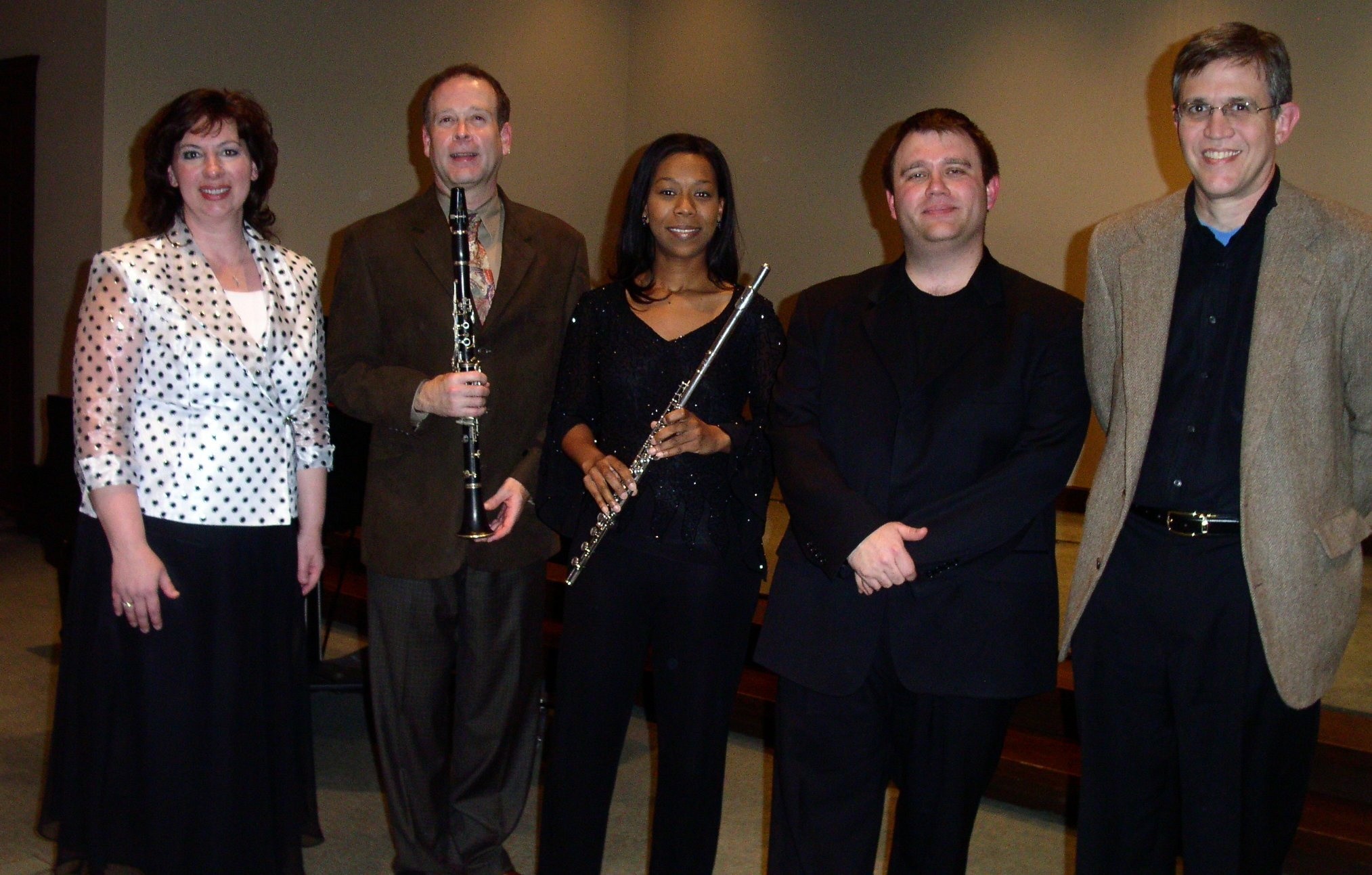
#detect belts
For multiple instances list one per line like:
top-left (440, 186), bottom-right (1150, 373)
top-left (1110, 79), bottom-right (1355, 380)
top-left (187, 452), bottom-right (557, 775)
top-left (1129, 503), bottom-right (1240, 540)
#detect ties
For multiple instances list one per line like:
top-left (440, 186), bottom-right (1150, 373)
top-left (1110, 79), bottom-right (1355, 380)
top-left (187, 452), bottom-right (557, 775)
top-left (466, 211), bottom-right (496, 325)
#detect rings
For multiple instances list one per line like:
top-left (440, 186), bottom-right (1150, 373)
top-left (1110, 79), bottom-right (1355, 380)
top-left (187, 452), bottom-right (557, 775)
top-left (122, 602), bottom-right (133, 609)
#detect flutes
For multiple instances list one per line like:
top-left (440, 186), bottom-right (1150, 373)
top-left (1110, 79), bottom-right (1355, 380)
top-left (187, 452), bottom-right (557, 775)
top-left (564, 261), bottom-right (773, 590)
top-left (446, 183), bottom-right (496, 540)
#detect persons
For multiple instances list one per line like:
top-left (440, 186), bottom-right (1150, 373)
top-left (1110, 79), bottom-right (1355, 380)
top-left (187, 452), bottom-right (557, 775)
top-left (536, 132), bottom-right (778, 875)
top-left (1059, 22), bottom-right (1371, 873)
top-left (325, 63), bottom-right (589, 874)
top-left (46, 85), bottom-right (338, 875)
top-left (766, 109), bottom-right (1093, 874)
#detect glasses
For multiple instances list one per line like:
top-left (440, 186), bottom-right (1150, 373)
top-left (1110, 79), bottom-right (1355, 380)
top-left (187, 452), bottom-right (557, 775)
top-left (1174, 100), bottom-right (1281, 120)
